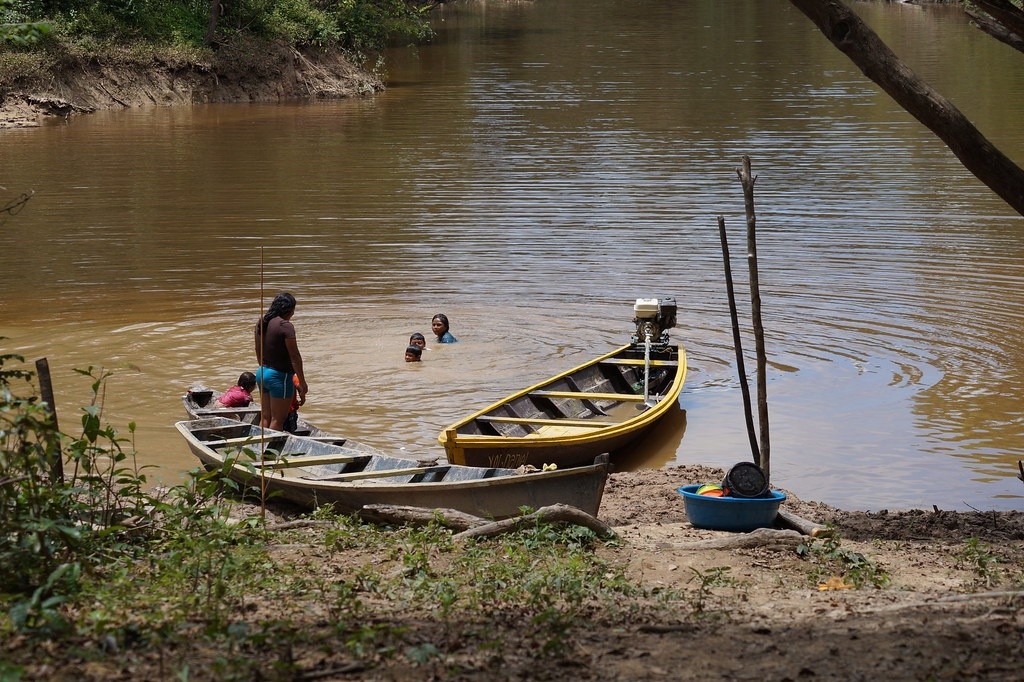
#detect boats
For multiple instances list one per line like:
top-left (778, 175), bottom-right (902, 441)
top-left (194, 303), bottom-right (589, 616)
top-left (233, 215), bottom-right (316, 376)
top-left (172, 380), bottom-right (609, 551)
top-left (437, 297), bottom-right (688, 478)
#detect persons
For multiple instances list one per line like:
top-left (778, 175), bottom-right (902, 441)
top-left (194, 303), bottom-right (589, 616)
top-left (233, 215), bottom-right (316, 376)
top-left (212, 373), bottom-right (256, 421)
top-left (410, 332), bottom-right (425, 349)
top-left (432, 314), bottom-right (457, 343)
top-left (255, 293), bottom-right (308, 431)
top-left (405, 344), bottom-right (422, 362)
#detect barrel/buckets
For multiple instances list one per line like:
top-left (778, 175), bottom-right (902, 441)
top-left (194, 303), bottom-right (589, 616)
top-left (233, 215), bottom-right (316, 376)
top-left (720, 462), bottom-right (769, 498)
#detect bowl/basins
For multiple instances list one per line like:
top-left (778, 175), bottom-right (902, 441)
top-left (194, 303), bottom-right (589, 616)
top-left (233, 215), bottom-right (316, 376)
top-left (677, 484), bottom-right (787, 531)
